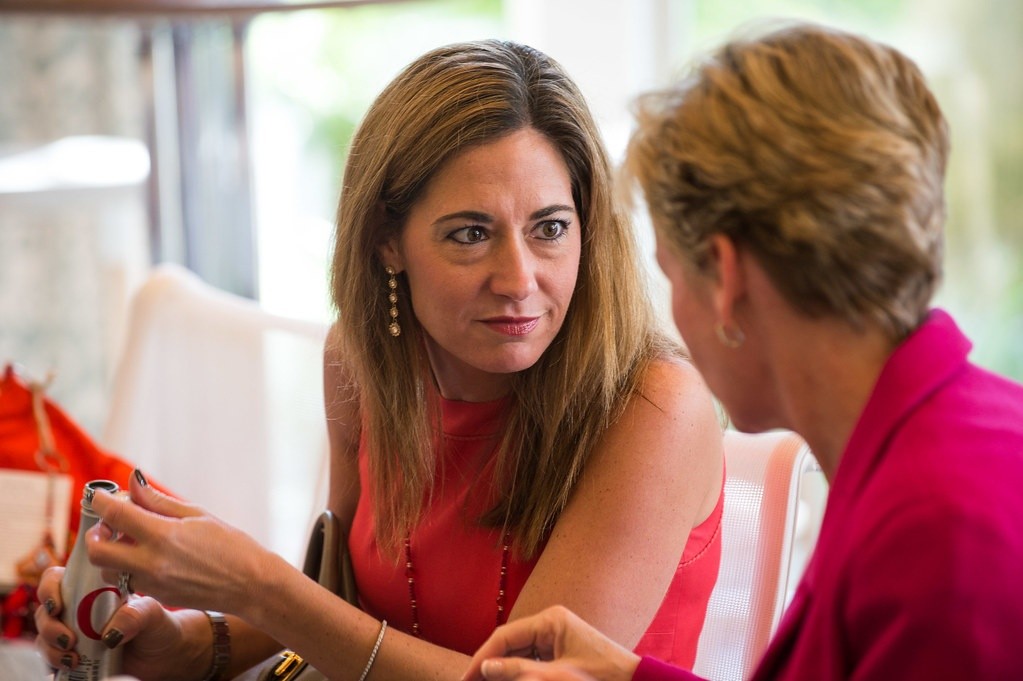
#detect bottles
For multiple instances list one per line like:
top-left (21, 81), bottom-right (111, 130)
top-left (48, 477), bottom-right (134, 680)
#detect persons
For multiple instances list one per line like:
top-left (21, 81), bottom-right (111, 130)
top-left (464, 22), bottom-right (1022, 681)
top-left (31, 38), bottom-right (724, 680)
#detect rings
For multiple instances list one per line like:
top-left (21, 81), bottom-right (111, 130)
top-left (118, 572), bottom-right (134, 594)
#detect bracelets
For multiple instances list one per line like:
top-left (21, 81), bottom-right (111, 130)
top-left (360, 620), bottom-right (387, 680)
top-left (203, 609), bottom-right (233, 680)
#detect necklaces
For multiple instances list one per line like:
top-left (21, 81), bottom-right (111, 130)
top-left (404, 518), bottom-right (512, 637)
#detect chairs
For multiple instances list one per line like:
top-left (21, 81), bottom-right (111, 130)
top-left (96, 258), bottom-right (350, 579)
